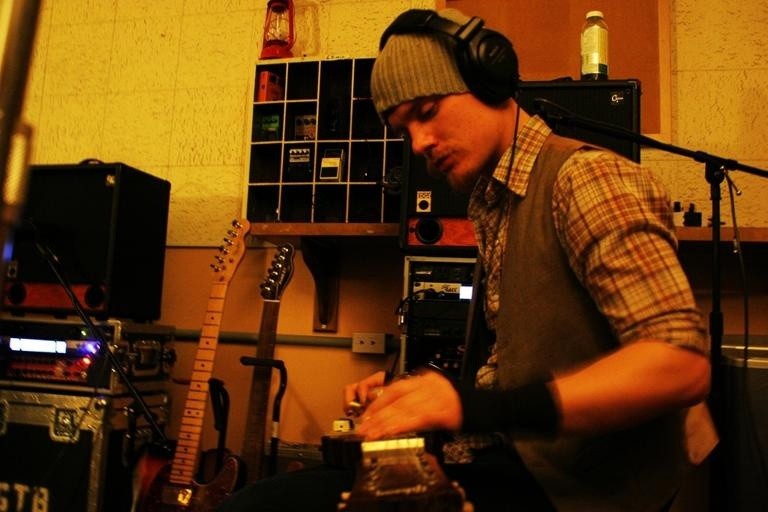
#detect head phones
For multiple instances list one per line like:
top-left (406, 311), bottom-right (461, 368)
top-left (378, 9), bottom-right (519, 106)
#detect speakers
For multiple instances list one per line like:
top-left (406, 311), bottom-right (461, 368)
top-left (399, 80), bottom-right (641, 257)
top-left (0, 165), bottom-right (170, 320)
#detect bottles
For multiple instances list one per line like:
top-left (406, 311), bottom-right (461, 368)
top-left (670, 200), bottom-right (702, 228)
top-left (578, 9), bottom-right (610, 82)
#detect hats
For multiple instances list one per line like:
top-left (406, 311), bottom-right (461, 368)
top-left (370, 8), bottom-right (485, 127)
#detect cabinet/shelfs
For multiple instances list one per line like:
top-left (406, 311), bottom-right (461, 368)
top-left (239, 56), bottom-right (405, 236)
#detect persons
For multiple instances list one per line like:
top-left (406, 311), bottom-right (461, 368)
top-left (341, 1), bottom-right (720, 511)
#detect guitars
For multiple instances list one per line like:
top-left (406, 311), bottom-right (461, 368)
top-left (319, 392), bottom-right (478, 512)
top-left (142, 220), bottom-right (251, 511)
